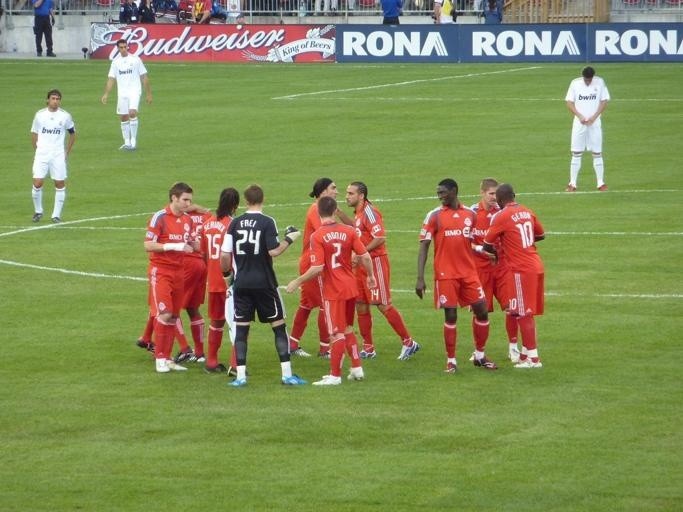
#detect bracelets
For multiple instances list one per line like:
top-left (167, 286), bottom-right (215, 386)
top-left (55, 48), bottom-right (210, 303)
top-left (475, 245), bottom-right (484, 253)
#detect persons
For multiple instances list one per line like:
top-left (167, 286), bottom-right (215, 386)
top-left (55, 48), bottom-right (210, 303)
top-left (415, 178), bottom-right (498, 373)
top-left (31, 0), bottom-right (56, 56)
top-left (564, 65), bottom-right (611, 193)
top-left (483, 183), bottom-right (546, 368)
top-left (284, 178), bottom-right (419, 386)
top-left (0, 0), bottom-right (504, 25)
top-left (135, 182), bottom-right (247, 378)
top-left (31, 88), bottom-right (76, 225)
top-left (218, 185), bottom-right (307, 387)
top-left (101, 39), bottom-right (154, 151)
top-left (470, 178), bottom-right (520, 364)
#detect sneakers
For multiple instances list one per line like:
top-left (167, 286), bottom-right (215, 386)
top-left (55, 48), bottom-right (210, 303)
top-left (118, 144), bottom-right (137, 150)
top-left (33, 213), bottom-right (43, 222)
top-left (52, 217), bottom-right (61, 223)
top-left (598, 185), bottom-right (609, 192)
top-left (564, 184), bottom-right (576, 192)
